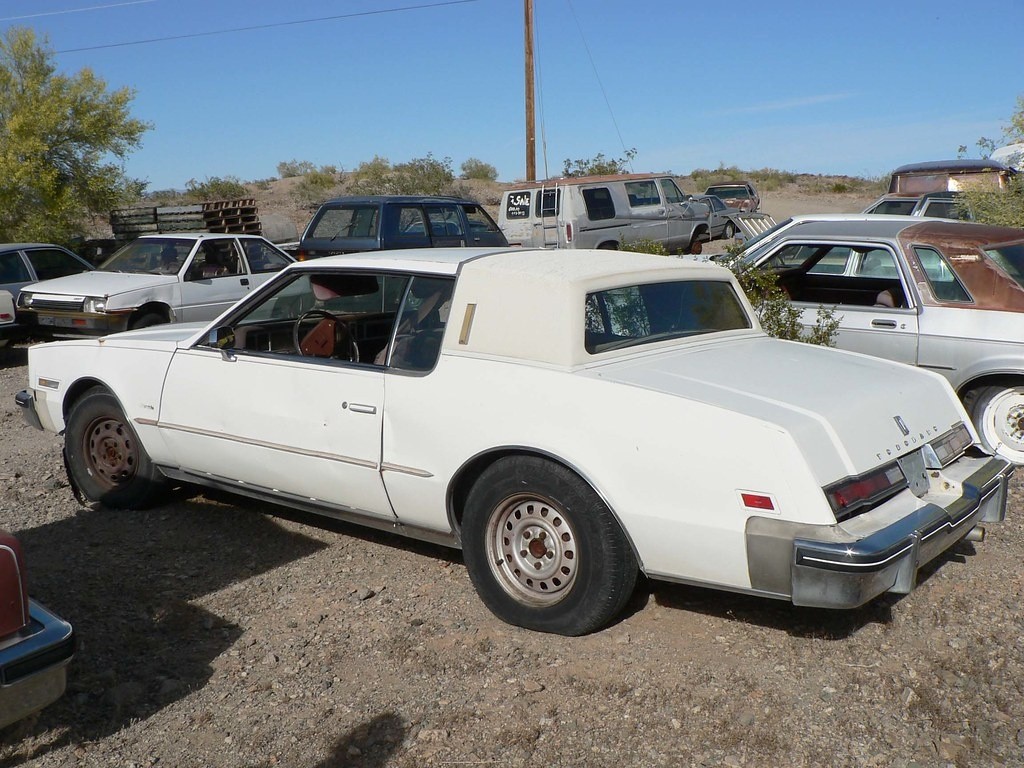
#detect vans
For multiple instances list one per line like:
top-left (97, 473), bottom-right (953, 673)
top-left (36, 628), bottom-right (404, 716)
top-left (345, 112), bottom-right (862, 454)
top-left (888, 158), bottom-right (1023, 194)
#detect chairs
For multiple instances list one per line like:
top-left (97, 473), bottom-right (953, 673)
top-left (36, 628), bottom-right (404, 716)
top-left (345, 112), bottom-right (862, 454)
top-left (197, 251), bottom-right (229, 279)
top-left (374, 287), bottom-right (448, 368)
top-left (873, 285), bottom-right (906, 308)
top-left (160, 249), bottom-right (178, 265)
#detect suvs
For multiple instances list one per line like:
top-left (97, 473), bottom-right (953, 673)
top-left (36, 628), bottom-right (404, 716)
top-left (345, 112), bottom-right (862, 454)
top-left (497, 174), bottom-right (713, 262)
top-left (281, 198), bottom-right (513, 264)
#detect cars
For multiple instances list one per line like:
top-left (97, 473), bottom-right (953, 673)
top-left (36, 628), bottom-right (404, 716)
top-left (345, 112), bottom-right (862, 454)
top-left (667, 223), bottom-right (1024, 470)
top-left (404, 220), bottom-right (462, 238)
top-left (687, 195), bottom-right (744, 240)
top-left (2, 234), bottom-right (326, 342)
top-left (702, 181), bottom-right (762, 228)
top-left (14, 245), bottom-right (1015, 640)
top-left (728, 195), bottom-right (1024, 283)
top-left (1, 244), bottom-right (97, 334)
